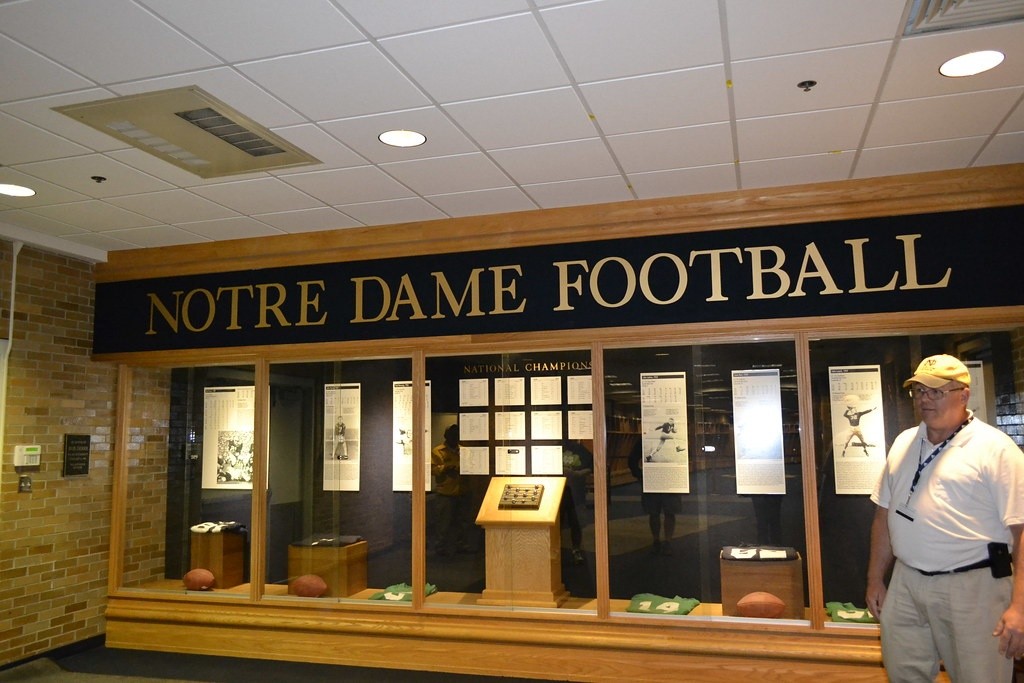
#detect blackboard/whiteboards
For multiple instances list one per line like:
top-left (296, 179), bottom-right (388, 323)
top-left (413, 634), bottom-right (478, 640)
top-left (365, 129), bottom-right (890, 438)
top-left (64, 433), bottom-right (90, 477)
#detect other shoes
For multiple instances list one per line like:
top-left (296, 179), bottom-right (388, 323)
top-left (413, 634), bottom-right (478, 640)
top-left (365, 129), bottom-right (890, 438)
top-left (653, 541), bottom-right (661, 553)
top-left (573, 548), bottom-right (584, 566)
top-left (435, 541), bottom-right (450, 555)
top-left (676, 446), bottom-right (685, 452)
top-left (646, 455), bottom-right (651, 461)
top-left (864, 450), bottom-right (869, 457)
top-left (455, 542), bottom-right (473, 552)
top-left (662, 540), bottom-right (673, 556)
top-left (843, 451), bottom-right (845, 457)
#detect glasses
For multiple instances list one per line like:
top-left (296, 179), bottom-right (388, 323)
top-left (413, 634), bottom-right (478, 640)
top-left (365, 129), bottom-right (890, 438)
top-left (909, 384), bottom-right (965, 400)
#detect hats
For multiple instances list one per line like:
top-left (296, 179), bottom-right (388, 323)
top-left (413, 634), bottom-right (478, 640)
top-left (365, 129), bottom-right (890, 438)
top-left (903, 353), bottom-right (971, 388)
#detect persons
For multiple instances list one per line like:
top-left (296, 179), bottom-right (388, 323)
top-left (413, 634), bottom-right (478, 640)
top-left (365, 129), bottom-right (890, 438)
top-left (555, 425), bottom-right (594, 566)
top-left (628, 437), bottom-right (682, 557)
top-left (742, 494), bottom-right (784, 546)
top-left (817, 438), bottom-right (896, 610)
top-left (431, 425), bottom-right (484, 561)
top-left (645, 417), bottom-right (686, 463)
top-left (217, 434), bottom-right (254, 482)
top-left (332, 416), bottom-right (348, 460)
top-left (866, 353), bottom-right (1024, 683)
top-left (842, 405), bottom-right (877, 458)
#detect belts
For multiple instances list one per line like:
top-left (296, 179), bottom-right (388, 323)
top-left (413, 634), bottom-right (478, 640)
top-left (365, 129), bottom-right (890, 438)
top-left (917, 553), bottom-right (1013, 576)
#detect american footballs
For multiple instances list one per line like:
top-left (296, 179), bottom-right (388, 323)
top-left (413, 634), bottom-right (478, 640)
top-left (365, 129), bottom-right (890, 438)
top-left (182, 568), bottom-right (216, 590)
top-left (735, 591), bottom-right (786, 618)
top-left (291, 574), bottom-right (328, 598)
